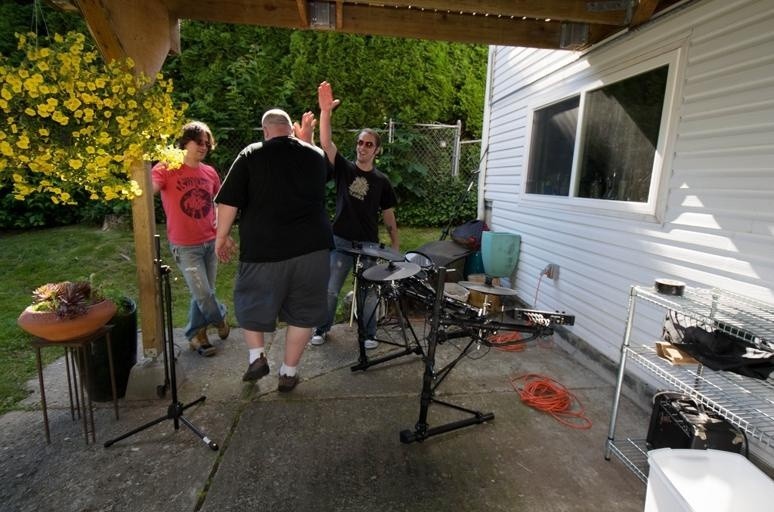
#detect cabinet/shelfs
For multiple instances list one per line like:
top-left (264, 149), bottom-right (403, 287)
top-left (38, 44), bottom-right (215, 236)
top-left (604, 284), bottom-right (774, 484)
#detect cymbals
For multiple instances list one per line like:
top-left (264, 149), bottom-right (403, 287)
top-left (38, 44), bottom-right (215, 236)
top-left (459, 281), bottom-right (519, 296)
top-left (361, 242), bottom-right (405, 263)
top-left (363, 262), bottom-right (421, 280)
top-left (339, 246), bottom-right (381, 260)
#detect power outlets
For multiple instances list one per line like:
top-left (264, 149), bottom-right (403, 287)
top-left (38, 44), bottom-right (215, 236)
top-left (547, 263), bottom-right (559, 280)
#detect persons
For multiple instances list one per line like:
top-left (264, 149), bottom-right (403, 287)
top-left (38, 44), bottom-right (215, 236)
top-left (151, 121), bottom-right (231, 356)
top-left (212, 108), bottom-right (333, 392)
top-left (311, 79), bottom-right (400, 349)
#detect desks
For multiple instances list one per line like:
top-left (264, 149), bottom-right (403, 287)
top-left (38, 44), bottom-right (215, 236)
top-left (31, 324), bottom-right (119, 445)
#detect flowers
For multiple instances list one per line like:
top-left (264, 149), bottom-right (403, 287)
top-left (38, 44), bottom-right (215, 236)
top-left (0, 32), bottom-right (189, 205)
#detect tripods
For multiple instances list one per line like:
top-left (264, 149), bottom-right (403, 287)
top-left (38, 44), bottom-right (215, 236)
top-left (103, 262), bottom-right (220, 454)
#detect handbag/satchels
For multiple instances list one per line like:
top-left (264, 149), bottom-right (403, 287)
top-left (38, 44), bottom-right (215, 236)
top-left (669, 324), bottom-right (774, 380)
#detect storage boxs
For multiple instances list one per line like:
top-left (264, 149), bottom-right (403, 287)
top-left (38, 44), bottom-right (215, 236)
top-left (645, 448), bottom-right (773, 511)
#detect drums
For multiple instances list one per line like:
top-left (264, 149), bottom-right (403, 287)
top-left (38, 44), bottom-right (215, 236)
top-left (405, 252), bottom-right (434, 271)
top-left (467, 287), bottom-right (503, 312)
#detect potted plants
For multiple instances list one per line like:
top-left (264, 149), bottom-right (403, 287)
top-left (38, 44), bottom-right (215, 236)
top-left (17, 280), bottom-right (117, 341)
top-left (71, 289), bottom-right (138, 401)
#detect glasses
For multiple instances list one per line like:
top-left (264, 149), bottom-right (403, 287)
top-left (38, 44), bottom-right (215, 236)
top-left (357, 140), bottom-right (374, 148)
top-left (193, 138), bottom-right (211, 149)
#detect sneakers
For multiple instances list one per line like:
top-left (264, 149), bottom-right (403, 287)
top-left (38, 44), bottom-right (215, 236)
top-left (214, 306), bottom-right (230, 340)
top-left (311, 328), bottom-right (327, 346)
top-left (243, 352), bottom-right (269, 382)
top-left (188, 328), bottom-right (217, 357)
top-left (278, 369), bottom-right (298, 393)
top-left (364, 336), bottom-right (379, 349)
